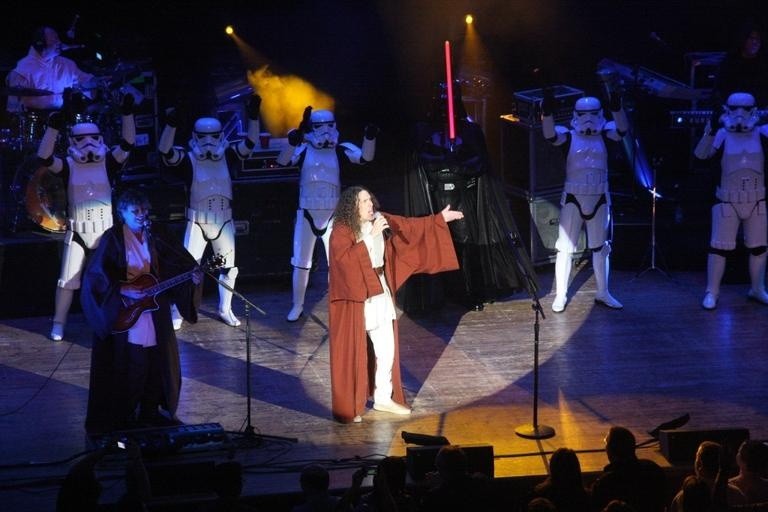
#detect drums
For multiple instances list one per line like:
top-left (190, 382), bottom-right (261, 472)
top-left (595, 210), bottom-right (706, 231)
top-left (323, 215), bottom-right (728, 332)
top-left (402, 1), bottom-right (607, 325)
top-left (71, 111), bottom-right (100, 125)
top-left (17, 113), bottom-right (42, 140)
top-left (111, 83), bottom-right (144, 112)
top-left (11, 158), bottom-right (70, 232)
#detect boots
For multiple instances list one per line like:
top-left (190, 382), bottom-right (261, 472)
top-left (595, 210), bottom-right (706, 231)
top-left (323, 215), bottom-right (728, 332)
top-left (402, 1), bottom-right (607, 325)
top-left (216, 267), bottom-right (242, 327)
top-left (703, 253), bottom-right (726, 310)
top-left (748, 251), bottom-right (768, 305)
top-left (552, 252), bottom-right (572, 313)
top-left (592, 241), bottom-right (624, 310)
top-left (51, 287), bottom-right (75, 342)
top-left (287, 267), bottom-right (310, 322)
top-left (171, 303), bottom-right (184, 331)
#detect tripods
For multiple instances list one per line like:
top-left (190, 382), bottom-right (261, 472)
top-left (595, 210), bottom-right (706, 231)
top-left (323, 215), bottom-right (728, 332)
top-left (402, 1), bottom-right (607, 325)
top-left (226, 310), bottom-right (299, 452)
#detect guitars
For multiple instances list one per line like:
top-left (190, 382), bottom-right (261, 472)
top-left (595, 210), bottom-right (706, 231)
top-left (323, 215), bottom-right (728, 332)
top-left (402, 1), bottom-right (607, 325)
top-left (108, 252), bottom-right (228, 332)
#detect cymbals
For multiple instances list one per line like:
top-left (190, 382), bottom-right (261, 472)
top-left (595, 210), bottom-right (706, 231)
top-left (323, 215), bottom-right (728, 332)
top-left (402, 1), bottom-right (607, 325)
top-left (57, 82), bottom-right (95, 95)
top-left (2, 84), bottom-right (53, 97)
top-left (107, 60), bottom-right (138, 75)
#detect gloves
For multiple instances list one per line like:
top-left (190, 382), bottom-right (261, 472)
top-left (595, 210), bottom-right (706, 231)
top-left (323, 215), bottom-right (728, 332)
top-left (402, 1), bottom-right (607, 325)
top-left (48, 113), bottom-right (64, 132)
top-left (249, 95), bottom-right (261, 120)
top-left (610, 91), bottom-right (621, 112)
top-left (364, 123), bottom-right (380, 140)
top-left (288, 129), bottom-right (301, 147)
top-left (708, 106), bottom-right (726, 137)
top-left (118, 93), bottom-right (135, 115)
top-left (541, 89), bottom-right (555, 116)
top-left (166, 108), bottom-right (180, 128)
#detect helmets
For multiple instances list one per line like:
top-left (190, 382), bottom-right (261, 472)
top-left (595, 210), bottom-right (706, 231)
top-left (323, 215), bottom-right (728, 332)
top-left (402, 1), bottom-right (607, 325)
top-left (304, 110), bottom-right (340, 149)
top-left (66, 123), bottom-right (107, 164)
top-left (571, 98), bottom-right (606, 136)
top-left (719, 93), bottom-right (759, 132)
top-left (189, 118), bottom-right (230, 161)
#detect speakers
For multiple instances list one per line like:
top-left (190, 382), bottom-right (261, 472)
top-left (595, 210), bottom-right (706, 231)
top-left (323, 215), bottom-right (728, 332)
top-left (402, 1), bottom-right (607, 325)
top-left (401, 442), bottom-right (496, 481)
top-left (657, 425), bottom-right (753, 465)
top-left (119, 454), bottom-right (222, 504)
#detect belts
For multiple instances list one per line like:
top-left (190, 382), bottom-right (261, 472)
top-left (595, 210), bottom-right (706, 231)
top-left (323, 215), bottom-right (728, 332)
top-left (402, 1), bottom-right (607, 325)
top-left (376, 266), bottom-right (384, 276)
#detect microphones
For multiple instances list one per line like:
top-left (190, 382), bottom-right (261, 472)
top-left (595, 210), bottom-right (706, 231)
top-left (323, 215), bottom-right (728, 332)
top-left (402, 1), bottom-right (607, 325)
top-left (61, 43), bottom-right (87, 51)
top-left (373, 210), bottom-right (394, 239)
top-left (140, 217), bottom-right (156, 242)
top-left (65, 14), bottom-right (81, 43)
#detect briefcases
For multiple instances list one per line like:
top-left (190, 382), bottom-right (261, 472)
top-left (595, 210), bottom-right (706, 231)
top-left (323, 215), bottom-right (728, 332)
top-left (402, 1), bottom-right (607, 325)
top-left (516, 85), bottom-right (585, 124)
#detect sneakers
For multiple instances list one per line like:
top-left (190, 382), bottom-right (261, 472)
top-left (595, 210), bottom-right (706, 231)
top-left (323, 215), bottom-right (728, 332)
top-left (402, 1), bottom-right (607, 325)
top-left (373, 400), bottom-right (411, 415)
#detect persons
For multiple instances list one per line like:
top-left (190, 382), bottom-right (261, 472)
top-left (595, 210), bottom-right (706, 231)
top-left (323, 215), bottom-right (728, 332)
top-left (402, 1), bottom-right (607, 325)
top-left (33, 89), bottom-right (140, 343)
top-left (294, 446), bottom-right (489, 511)
top-left (692, 89), bottom-right (768, 310)
top-left (709, 17), bottom-right (768, 112)
top-left (4, 19), bottom-right (117, 122)
top-left (274, 104), bottom-right (380, 324)
top-left (526, 427), bottom-right (768, 511)
top-left (399, 77), bottom-right (543, 318)
top-left (152, 93), bottom-right (264, 333)
top-left (541, 89), bottom-right (632, 314)
top-left (60, 461), bottom-right (252, 511)
top-left (80, 186), bottom-right (206, 441)
top-left (321, 180), bottom-right (466, 424)
